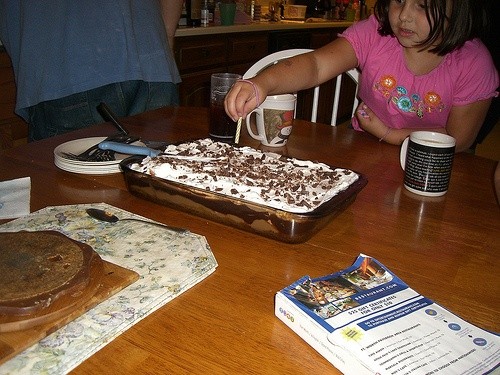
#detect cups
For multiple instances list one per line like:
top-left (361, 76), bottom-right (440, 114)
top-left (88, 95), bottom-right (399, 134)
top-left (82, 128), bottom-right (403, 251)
top-left (247, 93), bottom-right (298, 149)
top-left (399, 130), bottom-right (455, 197)
top-left (208, 72), bottom-right (241, 140)
top-left (219, 0), bottom-right (236, 25)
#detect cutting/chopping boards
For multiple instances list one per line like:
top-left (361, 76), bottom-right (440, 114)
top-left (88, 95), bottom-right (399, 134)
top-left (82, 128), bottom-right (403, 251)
top-left (0, 230), bottom-right (139, 366)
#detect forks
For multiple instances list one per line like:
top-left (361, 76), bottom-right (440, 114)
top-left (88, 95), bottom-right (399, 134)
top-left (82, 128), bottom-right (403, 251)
top-left (61, 132), bottom-right (140, 161)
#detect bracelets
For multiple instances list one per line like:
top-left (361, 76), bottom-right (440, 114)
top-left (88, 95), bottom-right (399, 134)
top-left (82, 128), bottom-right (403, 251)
top-left (231, 80), bottom-right (260, 108)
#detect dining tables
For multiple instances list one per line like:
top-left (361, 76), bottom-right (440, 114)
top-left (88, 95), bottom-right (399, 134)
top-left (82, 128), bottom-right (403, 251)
top-left (0, 104), bottom-right (499, 375)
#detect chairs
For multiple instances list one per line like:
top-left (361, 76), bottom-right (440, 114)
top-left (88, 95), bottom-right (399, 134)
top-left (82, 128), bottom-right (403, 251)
top-left (239, 44), bottom-right (368, 122)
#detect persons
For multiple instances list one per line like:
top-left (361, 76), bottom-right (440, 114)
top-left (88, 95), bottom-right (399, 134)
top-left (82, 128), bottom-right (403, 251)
top-left (1, 0), bottom-right (186, 146)
top-left (224, 0), bottom-right (499, 156)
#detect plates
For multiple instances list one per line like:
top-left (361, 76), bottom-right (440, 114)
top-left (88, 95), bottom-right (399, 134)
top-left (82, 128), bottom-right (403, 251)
top-left (119, 143), bottom-right (367, 245)
top-left (53, 136), bottom-right (145, 175)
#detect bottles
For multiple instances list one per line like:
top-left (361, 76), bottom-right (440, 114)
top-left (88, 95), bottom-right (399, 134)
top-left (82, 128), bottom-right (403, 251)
top-left (200, 0), bottom-right (214, 25)
top-left (308, 1), bottom-right (358, 22)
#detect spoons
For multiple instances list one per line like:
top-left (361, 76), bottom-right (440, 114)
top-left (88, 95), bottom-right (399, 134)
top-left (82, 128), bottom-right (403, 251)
top-left (85, 206), bottom-right (187, 234)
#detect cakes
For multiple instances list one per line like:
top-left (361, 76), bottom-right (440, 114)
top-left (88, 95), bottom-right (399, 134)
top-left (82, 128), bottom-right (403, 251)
top-left (126, 135), bottom-right (362, 245)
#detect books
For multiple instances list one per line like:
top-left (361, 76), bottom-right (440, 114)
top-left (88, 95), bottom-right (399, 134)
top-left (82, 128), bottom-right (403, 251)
top-left (272, 254), bottom-right (500, 375)
